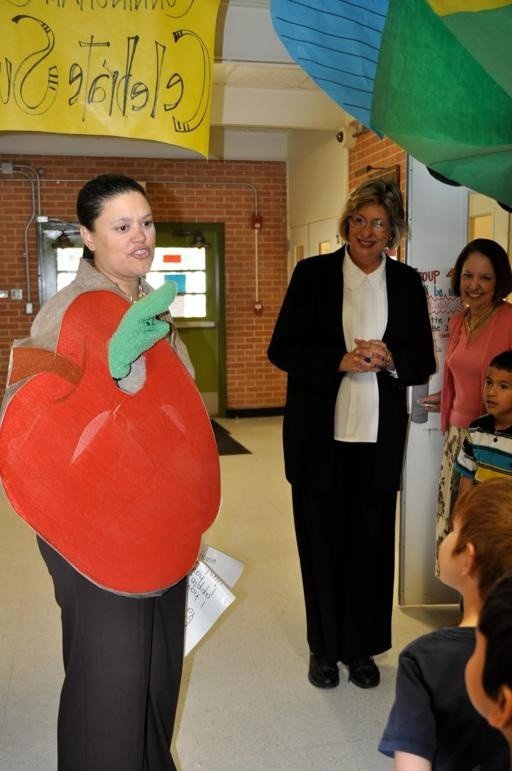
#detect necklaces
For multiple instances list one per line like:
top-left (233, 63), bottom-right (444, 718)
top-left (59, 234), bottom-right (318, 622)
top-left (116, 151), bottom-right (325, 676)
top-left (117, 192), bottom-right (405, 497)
top-left (464, 303), bottom-right (499, 348)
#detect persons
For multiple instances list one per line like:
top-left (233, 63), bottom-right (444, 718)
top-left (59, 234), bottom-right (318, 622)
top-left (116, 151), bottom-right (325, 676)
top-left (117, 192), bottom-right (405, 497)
top-left (265, 178), bottom-right (437, 690)
top-left (447, 350), bottom-right (512, 627)
top-left (2, 172), bottom-right (198, 770)
top-left (376, 478), bottom-right (511, 771)
top-left (416, 238), bottom-right (512, 610)
top-left (464, 571), bottom-right (512, 755)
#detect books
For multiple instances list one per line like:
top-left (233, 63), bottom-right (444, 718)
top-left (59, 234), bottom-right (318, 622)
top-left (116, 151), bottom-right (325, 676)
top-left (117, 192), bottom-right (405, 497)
top-left (199, 545), bottom-right (245, 591)
top-left (183, 561), bottom-right (237, 661)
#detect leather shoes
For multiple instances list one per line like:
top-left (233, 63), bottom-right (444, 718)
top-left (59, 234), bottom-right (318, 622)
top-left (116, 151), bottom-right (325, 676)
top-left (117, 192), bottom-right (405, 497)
top-left (308, 655), bottom-right (340, 689)
top-left (348, 656), bottom-right (381, 689)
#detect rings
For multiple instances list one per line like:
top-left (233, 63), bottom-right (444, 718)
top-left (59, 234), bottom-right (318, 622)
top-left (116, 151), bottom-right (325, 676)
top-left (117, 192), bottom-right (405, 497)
top-left (364, 357), bottom-right (371, 363)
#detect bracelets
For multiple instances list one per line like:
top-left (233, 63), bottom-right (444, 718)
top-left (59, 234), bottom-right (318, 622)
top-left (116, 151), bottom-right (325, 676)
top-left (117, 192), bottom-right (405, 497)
top-left (386, 352), bottom-right (392, 368)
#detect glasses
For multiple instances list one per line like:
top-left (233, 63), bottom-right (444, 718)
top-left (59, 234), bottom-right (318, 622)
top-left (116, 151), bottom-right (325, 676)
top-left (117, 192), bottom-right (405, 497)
top-left (347, 213), bottom-right (390, 231)
